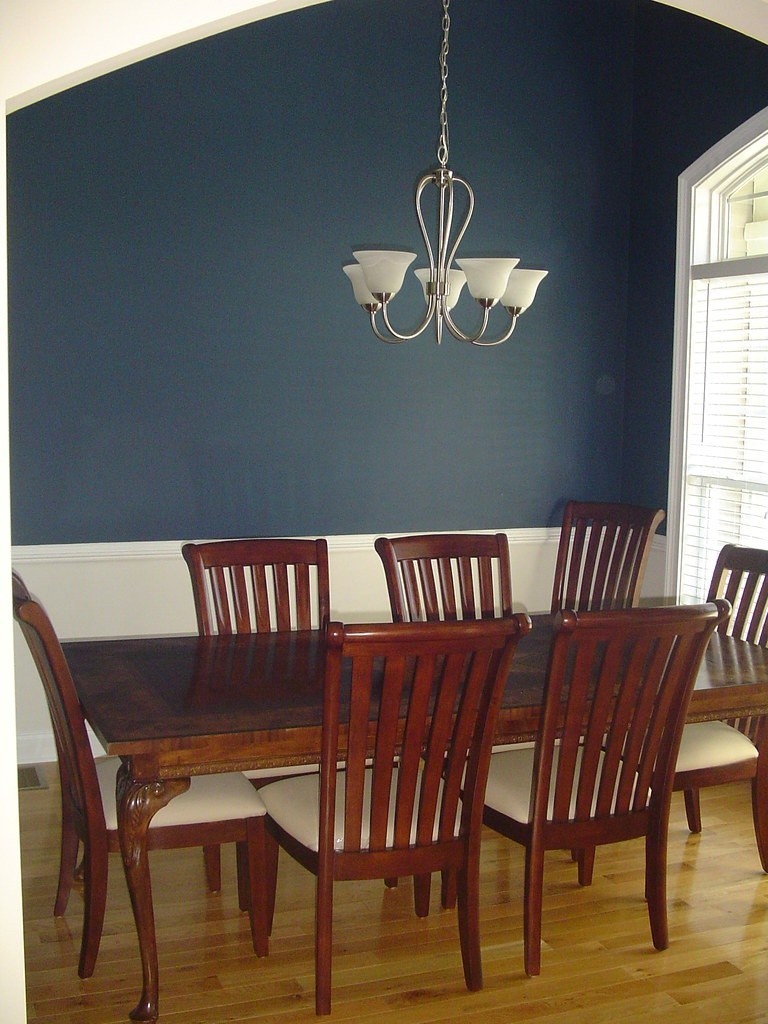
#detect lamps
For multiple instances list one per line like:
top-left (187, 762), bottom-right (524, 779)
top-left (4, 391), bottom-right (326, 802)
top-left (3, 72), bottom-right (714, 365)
top-left (342, 0), bottom-right (548, 346)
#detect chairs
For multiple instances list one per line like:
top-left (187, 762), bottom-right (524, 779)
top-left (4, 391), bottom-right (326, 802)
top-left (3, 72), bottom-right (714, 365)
top-left (458, 599), bottom-right (732, 972)
top-left (547, 499), bottom-right (665, 614)
top-left (374, 531), bottom-right (585, 758)
top-left (12, 571), bottom-right (271, 979)
top-left (594, 542), bottom-right (768, 873)
top-left (260, 611), bottom-right (533, 1017)
top-left (182, 538), bottom-right (400, 912)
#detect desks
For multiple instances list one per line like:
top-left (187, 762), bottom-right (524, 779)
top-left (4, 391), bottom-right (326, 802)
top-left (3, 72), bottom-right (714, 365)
top-left (63, 609), bottom-right (768, 1024)
